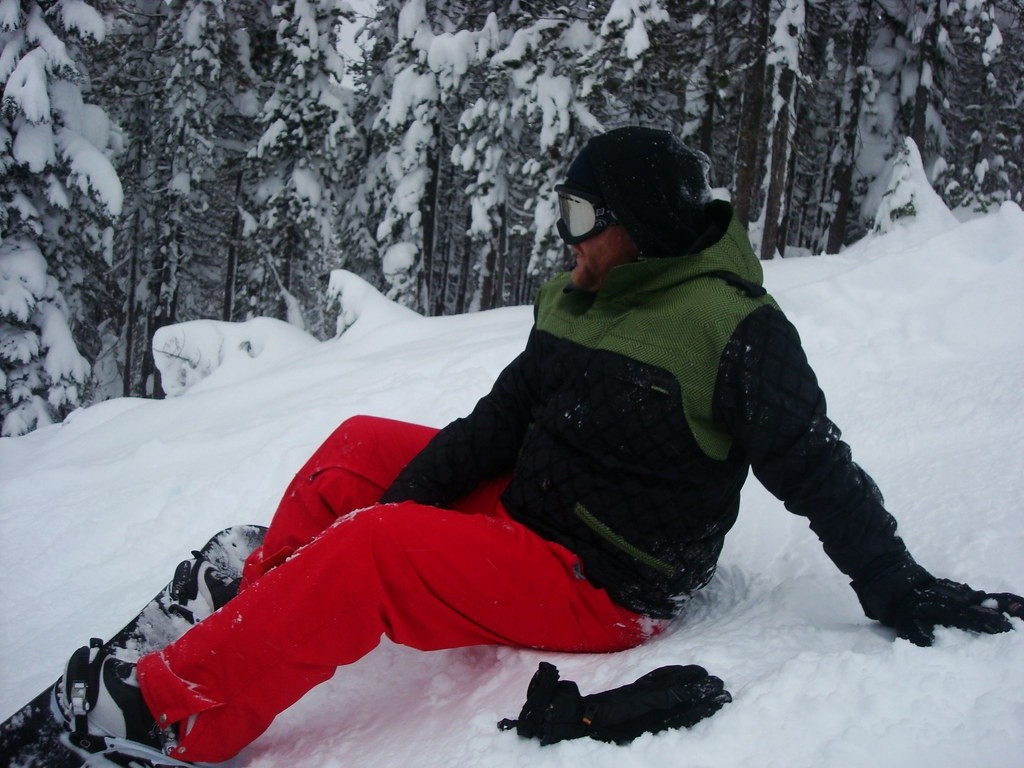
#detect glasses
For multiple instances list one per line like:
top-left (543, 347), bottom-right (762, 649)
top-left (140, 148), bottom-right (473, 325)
top-left (554, 184), bottom-right (620, 244)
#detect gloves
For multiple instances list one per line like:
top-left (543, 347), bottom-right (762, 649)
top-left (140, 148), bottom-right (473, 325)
top-left (850, 560), bottom-right (1024, 647)
top-left (500, 665), bottom-right (731, 746)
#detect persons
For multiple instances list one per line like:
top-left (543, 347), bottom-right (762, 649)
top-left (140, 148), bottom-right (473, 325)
top-left (61, 126), bottom-right (1024, 768)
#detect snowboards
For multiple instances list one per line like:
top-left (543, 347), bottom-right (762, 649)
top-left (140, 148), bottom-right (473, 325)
top-left (0, 523), bottom-right (267, 768)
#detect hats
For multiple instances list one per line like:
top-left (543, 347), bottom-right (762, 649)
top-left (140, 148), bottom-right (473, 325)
top-left (565, 127), bottom-right (733, 258)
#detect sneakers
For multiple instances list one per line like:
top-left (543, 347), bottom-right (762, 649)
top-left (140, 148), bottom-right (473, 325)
top-left (65, 646), bottom-right (183, 759)
top-left (167, 554), bottom-right (241, 627)
